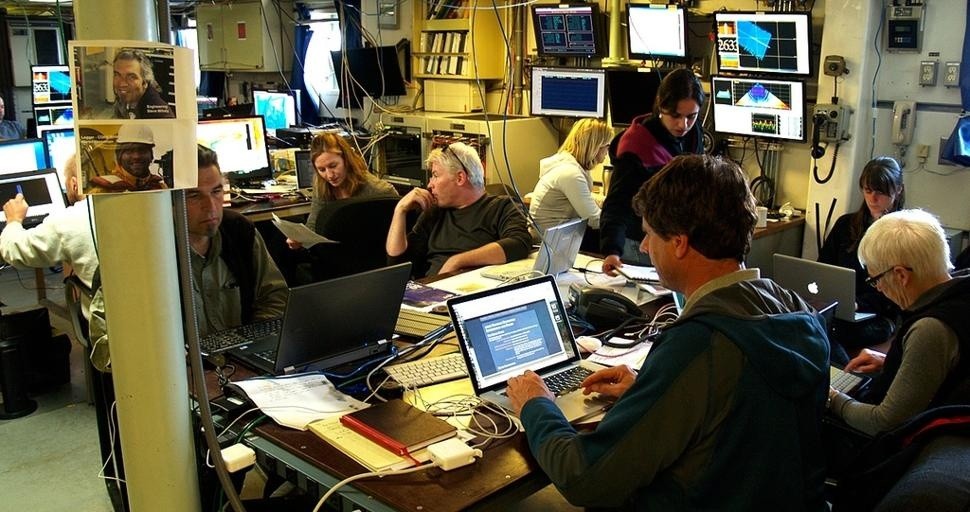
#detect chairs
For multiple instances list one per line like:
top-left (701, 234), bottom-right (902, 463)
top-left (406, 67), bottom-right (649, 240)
top-left (485, 183), bottom-right (517, 196)
top-left (877, 437), bottom-right (970, 511)
top-left (62, 263), bottom-right (102, 405)
top-left (309, 195), bottom-right (432, 280)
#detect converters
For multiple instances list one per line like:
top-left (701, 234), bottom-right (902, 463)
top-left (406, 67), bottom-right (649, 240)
top-left (427, 437), bottom-right (475, 471)
top-left (221, 443), bottom-right (256, 473)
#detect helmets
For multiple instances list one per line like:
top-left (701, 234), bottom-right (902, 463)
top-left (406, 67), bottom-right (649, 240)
top-left (116, 124), bottom-right (155, 147)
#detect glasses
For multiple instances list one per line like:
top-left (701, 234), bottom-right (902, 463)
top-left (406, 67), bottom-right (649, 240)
top-left (866, 266), bottom-right (912, 289)
top-left (433, 139), bottom-right (470, 176)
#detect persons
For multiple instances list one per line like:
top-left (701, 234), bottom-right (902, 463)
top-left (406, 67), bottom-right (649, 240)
top-left (87, 143), bottom-right (290, 374)
top-left (86, 124), bottom-right (169, 192)
top-left (112, 49), bottom-right (175, 119)
top-left (0, 93), bottom-right (27, 140)
top-left (598, 69), bottom-right (706, 278)
top-left (815, 157), bottom-right (907, 313)
top-left (387, 143), bottom-right (533, 284)
top-left (827, 208), bottom-right (970, 484)
top-left (227, 96), bottom-right (237, 106)
top-left (0, 155), bottom-right (98, 342)
top-left (287, 133), bottom-right (399, 263)
top-left (526, 119), bottom-right (616, 247)
top-left (505, 153), bottom-right (831, 511)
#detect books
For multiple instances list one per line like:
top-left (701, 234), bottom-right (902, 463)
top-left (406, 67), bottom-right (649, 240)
top-left (340, 398), bottom-right (459, 457)
top-left (306, 415), bottom-right (432, 478)
top-left (587, 336), bottom-right (653, 373)
top-left (418, 31), bottom-right (468, 75)
top-left (426, 1), bottom-right (470, 21)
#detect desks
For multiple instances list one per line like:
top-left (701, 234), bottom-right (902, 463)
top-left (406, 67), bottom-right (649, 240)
top-left (524, 192), bottom-right (806, 277)
top-left (188, 249), bottom-right (676, 511)
top-left (34, 198), bottom-right (312, 320)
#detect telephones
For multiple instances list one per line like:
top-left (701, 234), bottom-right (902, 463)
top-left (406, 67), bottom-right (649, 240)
top-left (890, 100), bottom-right (916, 169)
top-left (567, 283), bottom-right (659, 348)
top-left (208, 364), bottom-right (254, 425)
top-left (810, 102), bottom-right (855, 184)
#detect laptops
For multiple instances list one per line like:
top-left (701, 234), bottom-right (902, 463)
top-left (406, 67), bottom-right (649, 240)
top-left (446, 275), bottom-right (603, 416)
top-left (482, 219), bottom-right (589, 284)
top-left (224, 263), bottom-right (411, 376)
top-left (773, 254), bottom-right (879, 323)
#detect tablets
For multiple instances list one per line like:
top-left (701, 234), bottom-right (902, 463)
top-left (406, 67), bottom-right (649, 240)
top-left (828, 359), bottom-right (870, 409)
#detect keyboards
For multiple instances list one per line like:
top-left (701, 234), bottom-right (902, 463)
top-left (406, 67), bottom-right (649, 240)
top-left (386, 354), bottom-right (469, 392)
top-left (185, 316), bottom-right (283, 354)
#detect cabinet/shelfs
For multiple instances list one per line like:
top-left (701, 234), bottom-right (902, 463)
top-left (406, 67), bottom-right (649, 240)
top-left (412, 1), bottom-right (505, 79)
top-left (196, 3), bottom-right (282, 72)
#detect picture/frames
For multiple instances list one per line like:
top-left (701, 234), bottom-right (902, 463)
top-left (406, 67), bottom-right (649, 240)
top-left (68, 40), bottom-right (199, 195)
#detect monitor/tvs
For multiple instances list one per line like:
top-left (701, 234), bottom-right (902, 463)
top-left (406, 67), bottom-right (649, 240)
top-left (628, 6), bottom-right (686, 62)
top-left (1, 140), bottom-right (51, 178)
top-left (34, 107), bottom-right (74, 140)
top-left (2, 169), bottom-right (69, 236)
top-left (43, 128), bottom-right (80, 193)
top-left (714, 77), bottom-right (809, 146)
top-left (33, 68), bottom-right (73, 105)
top-left (254, 92), bottom-right (296, 139)
top-left (533, 6), bottom-right (600, 58)
top-left (331, 47), bottom-right (407, 98)
top-left (198, 116), bottom-right (273, 185)
top-left (716, 13), bottom-right (809, 74)
top-left (532, 67), bottom-right (606, 120)
